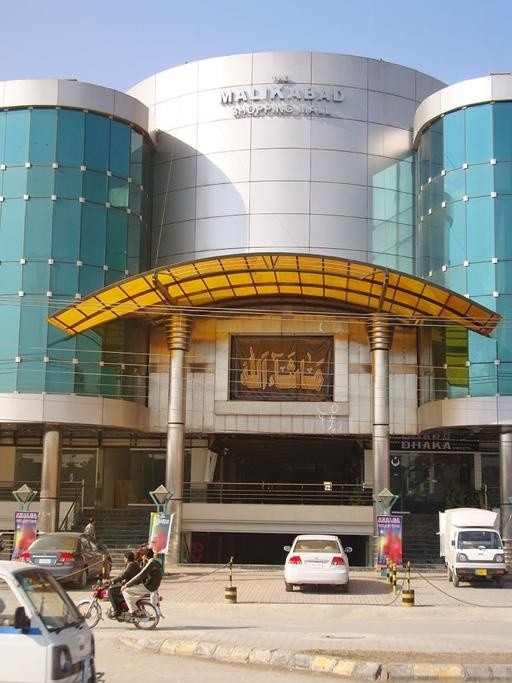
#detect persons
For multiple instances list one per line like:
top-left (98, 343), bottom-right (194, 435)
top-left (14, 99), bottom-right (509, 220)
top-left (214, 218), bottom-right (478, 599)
top-left (105, 549), bottom-right (162, 618)
top-left (83, 517), bottom-right (97, 542)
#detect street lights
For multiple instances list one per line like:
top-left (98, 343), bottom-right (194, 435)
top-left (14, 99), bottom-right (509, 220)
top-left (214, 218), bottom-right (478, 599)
top-left (148, 484), bottom-right (175, 576)
top-left (371, 486), bottom-right (399, 581)
top-left (11, 482), bottom-right (39, 512)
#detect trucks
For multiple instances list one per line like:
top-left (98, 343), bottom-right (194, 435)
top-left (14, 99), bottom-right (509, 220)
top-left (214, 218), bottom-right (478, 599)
top-left (437, 506), bottom-right (509, 588)
top-left (0, 558), bottom-right (100, 682)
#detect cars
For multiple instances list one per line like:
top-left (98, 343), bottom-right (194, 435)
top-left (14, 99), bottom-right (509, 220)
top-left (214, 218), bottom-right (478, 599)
top-left (12, 528), bottom-right (114, 591)
top-left (283, 532), bottom-right (355, 594)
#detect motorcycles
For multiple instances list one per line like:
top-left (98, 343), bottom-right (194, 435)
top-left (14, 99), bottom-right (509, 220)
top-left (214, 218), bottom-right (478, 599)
top-left (71, 579), bottom-right (164, 631)
